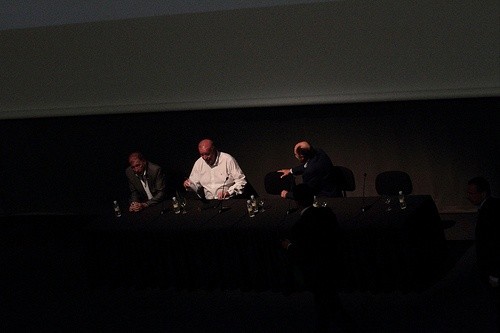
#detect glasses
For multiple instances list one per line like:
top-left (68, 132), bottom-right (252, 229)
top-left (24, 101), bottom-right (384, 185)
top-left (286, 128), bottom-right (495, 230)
top-left (200, 151), bottom-right (213, 158)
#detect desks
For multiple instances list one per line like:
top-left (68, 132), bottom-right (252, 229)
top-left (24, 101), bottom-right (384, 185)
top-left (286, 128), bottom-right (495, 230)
top-left (82, 194), bottom-right (450, 296)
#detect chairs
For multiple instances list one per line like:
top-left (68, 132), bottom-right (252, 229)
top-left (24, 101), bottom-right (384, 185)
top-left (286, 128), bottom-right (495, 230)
top-left (264, 171), bottom-right (293, 195)
top-left (374, 171), bottom-right (412, 196)
top-left (332, 165), bottom-right (355, 198)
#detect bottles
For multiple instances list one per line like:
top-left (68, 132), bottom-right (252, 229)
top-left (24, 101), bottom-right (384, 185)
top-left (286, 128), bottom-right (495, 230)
top-left (250, 194), bottom-right (258, 212)
top-left (172, 197), bottom-right (180, 214)
top-left (313, 195), bottom-right (319, 208)
top-left (399, 191), bottom-right (407, 209)
top-left (112, 201), bottom-right (121, 217)
top-left (247, 200), bottom-right (256, 218)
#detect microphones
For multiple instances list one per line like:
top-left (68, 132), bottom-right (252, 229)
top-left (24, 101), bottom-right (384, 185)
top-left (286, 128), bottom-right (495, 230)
top-left (217, 176), bottom-right (231, 214)
top-left (361, 173), bottom-right (368, 215)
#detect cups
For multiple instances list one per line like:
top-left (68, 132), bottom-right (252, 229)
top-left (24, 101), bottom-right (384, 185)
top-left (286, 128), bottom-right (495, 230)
top-left (179, 197), bottom-right (186, 214)
top-left (258, 200), bottom-right (265, 213)
top-left (321, 199), bottom-right (328, 207)
top-left (384, 194), bottom-right (393, 211)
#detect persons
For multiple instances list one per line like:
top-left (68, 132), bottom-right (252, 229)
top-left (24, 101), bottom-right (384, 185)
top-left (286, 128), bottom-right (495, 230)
top-left (124, 152), bottom-right (166, 213)
top-left (277, 183), bottom-right (346, 333)
top-left (277, 140), bottom-right (344, 201)
top-left (183, 139), bottom-right (248, 201)
top-left (464, 176), bottom-right (500, 332)
